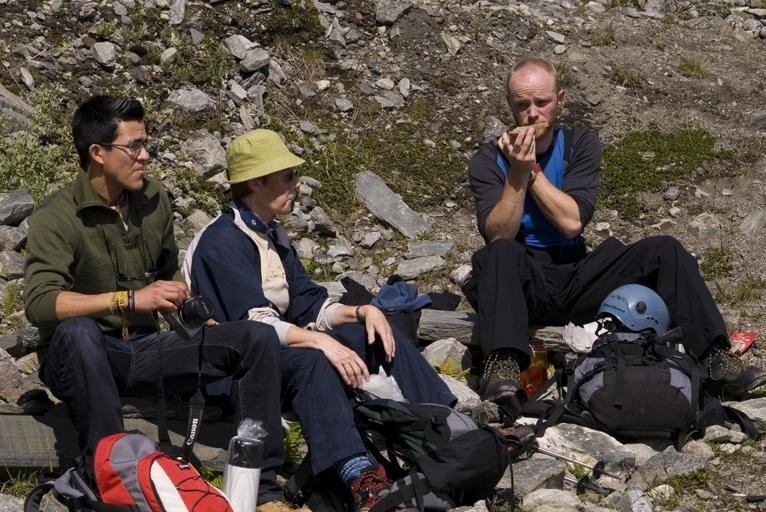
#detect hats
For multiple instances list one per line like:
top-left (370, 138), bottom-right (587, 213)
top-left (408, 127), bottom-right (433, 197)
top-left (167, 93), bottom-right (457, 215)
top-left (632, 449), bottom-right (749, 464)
top-left (369, 274), bottom-right (432, 316)
top-left (222, 128), bottom-right (306, 185)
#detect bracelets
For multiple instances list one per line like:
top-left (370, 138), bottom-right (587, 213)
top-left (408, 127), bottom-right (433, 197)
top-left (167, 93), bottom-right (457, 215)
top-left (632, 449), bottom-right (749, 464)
top-left (356, 304), bottom-right (362, 324)
top-left (107, 288), bottom-right (136, 315)
top-left (528, 162), bottom-right (542, 186)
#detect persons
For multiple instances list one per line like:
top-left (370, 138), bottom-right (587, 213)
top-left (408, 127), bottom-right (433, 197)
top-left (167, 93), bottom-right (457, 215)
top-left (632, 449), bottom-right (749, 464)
top-left (21, 94), bottom-right (312, 512)
top-left (179, 126), bottom-right (536, 511)
top-left (466, 59), bottom-right (765, 421)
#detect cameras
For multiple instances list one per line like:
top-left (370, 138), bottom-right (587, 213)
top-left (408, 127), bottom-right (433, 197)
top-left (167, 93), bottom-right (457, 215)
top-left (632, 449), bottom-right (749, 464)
top-left (162, 295), bottom-right (217, 336)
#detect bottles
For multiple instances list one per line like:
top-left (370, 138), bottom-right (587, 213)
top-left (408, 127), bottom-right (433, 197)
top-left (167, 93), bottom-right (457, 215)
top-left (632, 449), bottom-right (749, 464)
top-left (223, 437), bottom-right (265, 512)
top-left (520, 338), bottom-right (548, 401)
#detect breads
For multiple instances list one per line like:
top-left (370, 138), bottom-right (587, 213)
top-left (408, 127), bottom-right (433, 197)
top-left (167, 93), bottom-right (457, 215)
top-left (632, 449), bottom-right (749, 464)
top-left (508, 122), bottom-right (549, 137)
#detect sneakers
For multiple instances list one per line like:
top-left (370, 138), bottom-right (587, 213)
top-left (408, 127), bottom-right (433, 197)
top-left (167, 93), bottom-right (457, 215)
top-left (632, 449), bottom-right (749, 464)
top-left (255, 500), bottom-right (312, 512)
top-left (346, 462), bottom-right (421, 512)
top-left (703, 343), bottom-right (765, 394)
top-left (473, 351), bottom-right (524, 402)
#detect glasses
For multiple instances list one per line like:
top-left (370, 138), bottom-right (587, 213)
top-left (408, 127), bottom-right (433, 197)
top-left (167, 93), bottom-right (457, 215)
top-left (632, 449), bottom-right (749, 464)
top-left (272, 169), bottom-right (299, 182)
top-left (102, 137), bottom-right (153, 156)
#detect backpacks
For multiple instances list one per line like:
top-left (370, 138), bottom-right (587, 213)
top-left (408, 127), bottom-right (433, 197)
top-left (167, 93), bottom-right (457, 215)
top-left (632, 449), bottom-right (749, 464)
top-left (566, 332), bottom-right (702, 442)
top-left (346, 388), bottom-right (510, 507)
top-left (23, 429), bottom-right (233, 512)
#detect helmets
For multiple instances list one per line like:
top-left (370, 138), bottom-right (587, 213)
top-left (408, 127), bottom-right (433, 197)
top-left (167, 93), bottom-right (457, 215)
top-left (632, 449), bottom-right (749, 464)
top-left (595, 285), bottom-right (671, 338)
